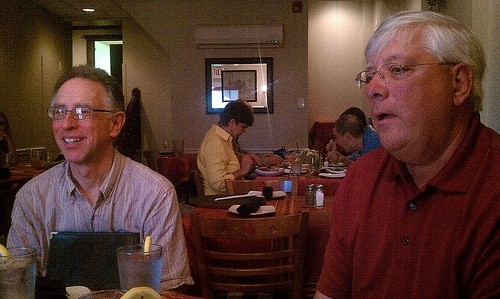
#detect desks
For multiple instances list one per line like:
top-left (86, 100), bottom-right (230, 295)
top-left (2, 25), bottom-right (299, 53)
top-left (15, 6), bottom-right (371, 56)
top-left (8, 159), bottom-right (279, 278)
top-left (158, 153), bottom-right (197, 187)
top-left (182, 198), bottom-right (333, 282)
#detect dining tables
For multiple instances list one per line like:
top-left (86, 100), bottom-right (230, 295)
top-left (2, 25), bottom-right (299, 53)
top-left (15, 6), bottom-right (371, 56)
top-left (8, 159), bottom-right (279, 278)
top-left (255, 173), bottom-right (344, 197)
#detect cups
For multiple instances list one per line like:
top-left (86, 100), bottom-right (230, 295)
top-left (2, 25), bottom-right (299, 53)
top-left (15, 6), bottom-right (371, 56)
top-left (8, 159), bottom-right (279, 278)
top-left (308, 150), bottom-right (321, 178)
top-left (0, 247), bottom-right (38, 299)
top-left (280, 181), bottom-right (293, 193)
top-left (290, 158), bottom-right (302, 175)
top-left (117, 242), bottom-right (163, 293)
top-left (172, 138), bottom-right (184, 157)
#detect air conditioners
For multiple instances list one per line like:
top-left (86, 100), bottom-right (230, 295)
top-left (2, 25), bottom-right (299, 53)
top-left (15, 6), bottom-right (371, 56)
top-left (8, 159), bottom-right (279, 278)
top-left (195, 23), bottom-right (284, 48)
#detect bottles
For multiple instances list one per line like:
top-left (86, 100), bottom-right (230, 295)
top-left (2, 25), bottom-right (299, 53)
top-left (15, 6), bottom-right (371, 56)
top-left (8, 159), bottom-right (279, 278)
top-left (304, 184), bottom-right (316, 206)
top-left (314, 184), bottom-right (325, 207)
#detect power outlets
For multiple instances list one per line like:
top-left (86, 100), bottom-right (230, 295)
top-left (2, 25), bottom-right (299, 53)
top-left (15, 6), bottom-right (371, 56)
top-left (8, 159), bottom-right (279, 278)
top-left (298, 98), bottom-right (304, 107)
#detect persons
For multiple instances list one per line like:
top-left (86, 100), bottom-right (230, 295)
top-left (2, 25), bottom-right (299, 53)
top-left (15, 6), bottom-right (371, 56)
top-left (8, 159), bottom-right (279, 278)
top-left (310, 9), bottom-right (500, 299)
top-left (0, 65), bottom-right (380, 299)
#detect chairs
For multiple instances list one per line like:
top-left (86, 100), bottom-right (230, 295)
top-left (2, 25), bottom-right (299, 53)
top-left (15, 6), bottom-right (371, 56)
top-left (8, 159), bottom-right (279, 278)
top-left (226, 177), bottom-right (299, 197)
top-left (189, 210), bottom-right (310, 299)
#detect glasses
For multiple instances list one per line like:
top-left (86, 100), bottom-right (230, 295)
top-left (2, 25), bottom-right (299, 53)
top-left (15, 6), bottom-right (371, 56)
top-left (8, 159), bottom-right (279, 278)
top-left (46, 105), bottom-right (113, 120)
top-left (355, 61), bottom-right (459, 89)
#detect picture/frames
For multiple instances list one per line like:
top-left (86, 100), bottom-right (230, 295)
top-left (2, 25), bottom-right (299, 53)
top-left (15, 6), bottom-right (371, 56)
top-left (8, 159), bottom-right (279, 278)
top-left (204, 57), bottom-right (273, 114)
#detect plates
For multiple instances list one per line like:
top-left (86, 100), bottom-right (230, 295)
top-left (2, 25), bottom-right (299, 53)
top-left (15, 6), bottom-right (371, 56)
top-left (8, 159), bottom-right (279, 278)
top-left (10, 170), bottom-right (25, 176)
top-left (74, 288), bottom-right (207, 299)
top-left (284, 158), bottom-right (347, 177)
top-left (228, 204), bottom-right (277, 216)
top-left (247, 190), bottom-right (287, 199)
